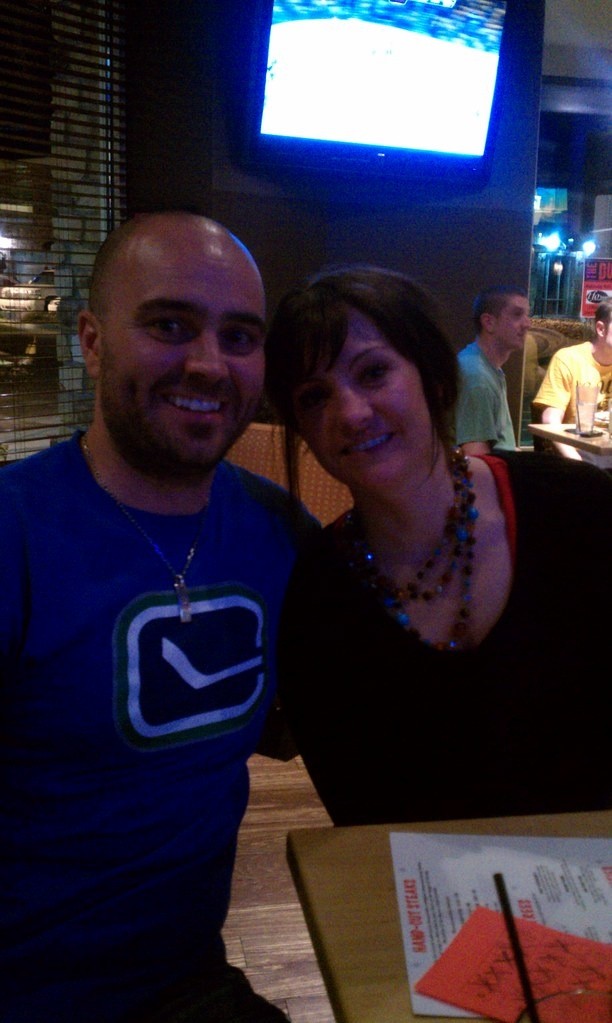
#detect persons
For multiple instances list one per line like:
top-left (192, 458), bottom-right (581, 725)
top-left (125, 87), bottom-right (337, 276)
top-left (440, 285), bottom-right (530, 452)
top-left (532, 299), bottom-right (612, 469)
top-left (278, 268), bottom-right (612, 830)
top-left (0, 209), bottom-right (324, 1023)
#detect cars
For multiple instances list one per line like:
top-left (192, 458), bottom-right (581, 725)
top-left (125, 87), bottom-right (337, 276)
top-left (531, 217), bottom-right (597, 280)
top-left (0, 265), bottom-right (61, 346)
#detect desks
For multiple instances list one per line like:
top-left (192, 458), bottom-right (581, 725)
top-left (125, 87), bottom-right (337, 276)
top-left (286, 811), bottom-right (612, 1023)
top-left (525, 424), bottom-right (612, 457)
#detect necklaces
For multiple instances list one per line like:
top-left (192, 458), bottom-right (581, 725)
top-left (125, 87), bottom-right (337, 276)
top-left (83, 431), bottom-right (209, 623)
top-left (341, 443), bottom-right (479, 651)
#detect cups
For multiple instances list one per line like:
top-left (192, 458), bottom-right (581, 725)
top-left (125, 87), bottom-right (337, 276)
top-left (575, 385), bottom-right (599, 434)
top-left (515, 989), bottom-right (612, 1023)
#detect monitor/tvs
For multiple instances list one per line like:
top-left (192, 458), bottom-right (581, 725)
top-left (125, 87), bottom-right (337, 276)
top-left (239, 0), bottom-right (521, 186)
